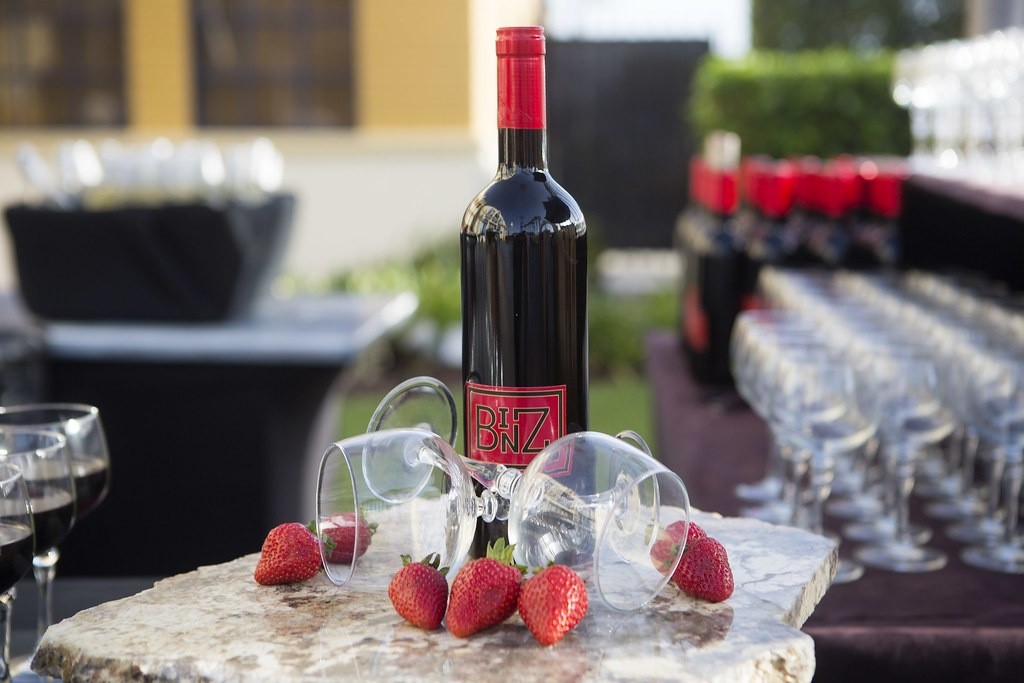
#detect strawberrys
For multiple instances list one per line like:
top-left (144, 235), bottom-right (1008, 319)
top-left (444, 557), bottom-right (523, 638)
top-left (388, 562), bottom-right (448, 630)
top-left (517, 561), bottom-right (590, 646)
top-left (316, 512), bottom-right (371, 563)
top-left (650, 520), bottom-right (736, 603)
top-left (253, 521), bottom-right (324, 586)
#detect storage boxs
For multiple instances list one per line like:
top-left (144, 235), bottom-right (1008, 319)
top-left (28, 296), bottom-right (430, 586)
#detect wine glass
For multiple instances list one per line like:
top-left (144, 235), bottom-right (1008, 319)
top-left (361, 375), bottom-right (691, 612)
top-left (0, 403), bottom-right (110, 683)
top-left (892, 23), bottom-right (1024, 192)
top-left (729, 264), bottom-right (1024, 585)
top-left (314, 430), bottom-right (661, 592)
top-left (0, 426), bottom-right (78, 683)
top-left (0, 460), bottom-right (36, 683)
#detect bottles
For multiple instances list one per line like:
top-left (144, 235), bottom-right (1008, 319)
top-left (460, 25), bottom-right (590, 573)
top-left (675, 128), bottom-right (910, 393)
top-left (15, 138), bottom-right (288, 214)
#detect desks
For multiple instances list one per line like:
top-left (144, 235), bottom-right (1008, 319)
top-left (640, 337), bottom-right (1024, 682)
